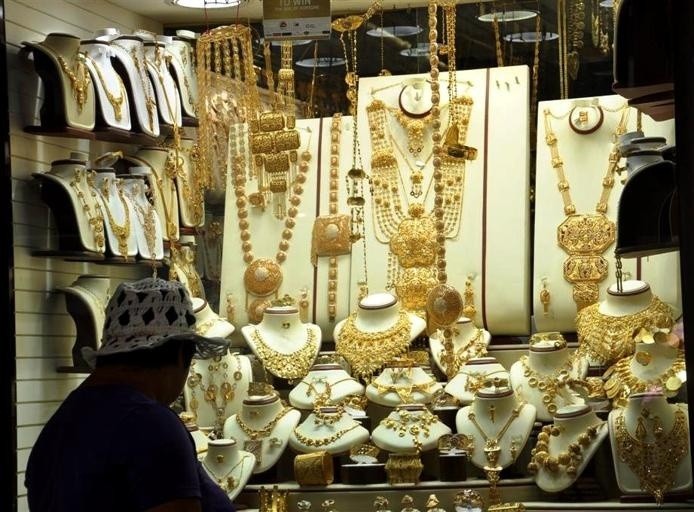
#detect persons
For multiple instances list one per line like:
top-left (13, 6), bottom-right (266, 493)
top-left (24, 278), bottom-right (236, 512)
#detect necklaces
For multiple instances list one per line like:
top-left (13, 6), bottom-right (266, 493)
top-left (47, 144), bottom-right (204, 277)
top-left (196, 315), bottom-right (220, 334)
top-left (70, 279), bottom-right (106, 318)
top-left (535, 420), bottom-right (606, 477)
top-left (252, 325), bottom-right (318, 385)
top-left (336, 310), bottom-right (411, 382)
top-left (166, 255), bottom-right (201, 298)
top-left (470, 402), bottom-right (525, 488)
top-left (577, 296), bottom-right (673, 365)
top-left (381, 409), bottom-right (440, 450)
top-left (545, 103), bottom-right (632, 313)
top-left (190, 358), bottom-right (241, 432)
top-left (197, 0), bottom-right (625, 318)
top-left (458, 369), bottom-right (508, 391)
top-left (521, 353), bottom-right (574, 413)
top-left (300, 378), bottom-right (356, 404)
top-left (203, 454), bottom-right (249, 494)
top-left (236, 406), bottom-right (293, 463)
top-left (438, 326), bottom-right (483, 371)
top-left (603, 355), bottom-right (687, 403)
top-left (294, 405), bottom-right (361, 446)
top-left (615, 405), bottom-right (688, 507)
top-left (39, 42), bottom-right (196, 143)
top-left (371, 361), bottom-right (437, 403)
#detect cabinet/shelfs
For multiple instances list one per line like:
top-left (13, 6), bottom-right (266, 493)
top-left (26, 115), bottom-right (205, 376)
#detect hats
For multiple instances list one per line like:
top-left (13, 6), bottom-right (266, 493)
top-left (80, 276), bottom-right (232, 370)
